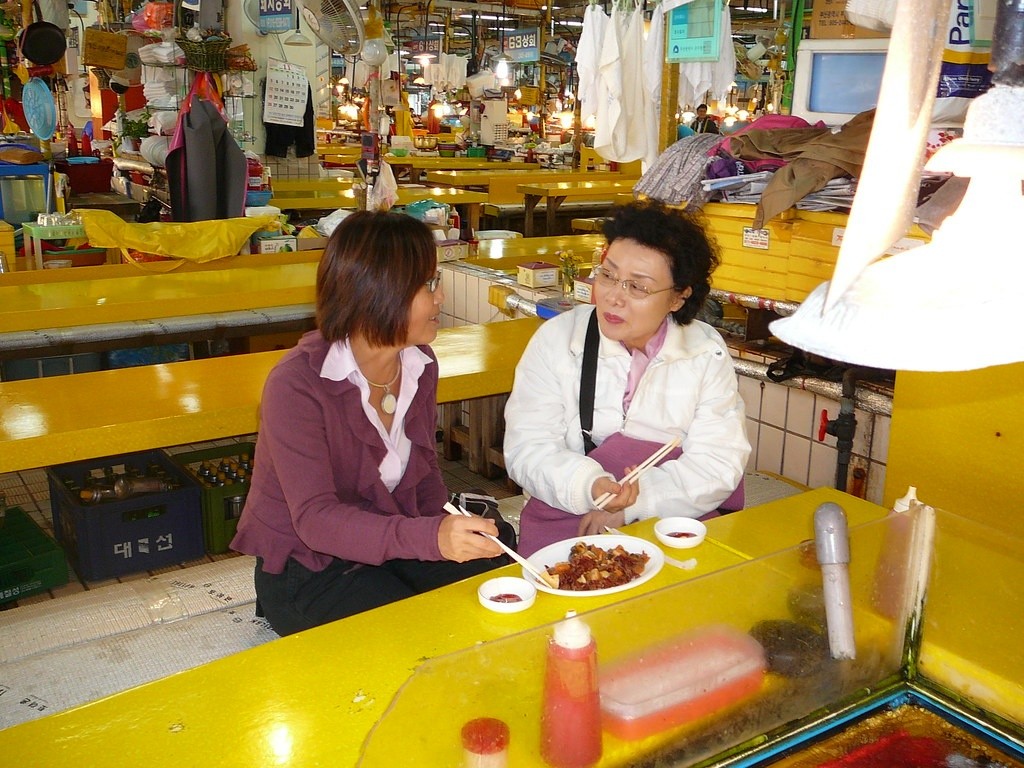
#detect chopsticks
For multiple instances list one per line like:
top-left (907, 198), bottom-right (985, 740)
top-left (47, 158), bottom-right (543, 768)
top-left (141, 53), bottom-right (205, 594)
top-left (443, 501), bottom-right (557, 588)
top-left (592, 438), bottom-right (682, 510)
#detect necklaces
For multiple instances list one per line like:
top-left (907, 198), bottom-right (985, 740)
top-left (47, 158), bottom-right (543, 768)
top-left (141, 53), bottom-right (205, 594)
top-left (368, 357), bottom-right (401, 415)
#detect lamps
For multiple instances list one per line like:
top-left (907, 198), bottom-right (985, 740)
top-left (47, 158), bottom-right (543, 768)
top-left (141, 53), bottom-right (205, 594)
top-left (432, 99), bottom-right (449, 118)
top-left (490, 2), bottom-right (514, 77)
top-left (413, 1), bottom-right (437, 65)
top-left (283, 0), bottom-right (312, 47)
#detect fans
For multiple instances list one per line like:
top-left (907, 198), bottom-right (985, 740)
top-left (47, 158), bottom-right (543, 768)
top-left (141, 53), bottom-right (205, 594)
top-left (243, 0), bottom-right (297, 38)
top-left (299, 0), bottom-right (362, 55)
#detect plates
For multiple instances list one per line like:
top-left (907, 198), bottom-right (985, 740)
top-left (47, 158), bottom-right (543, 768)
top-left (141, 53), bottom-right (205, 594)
top-left (522, 535), bottom-right (664, 594)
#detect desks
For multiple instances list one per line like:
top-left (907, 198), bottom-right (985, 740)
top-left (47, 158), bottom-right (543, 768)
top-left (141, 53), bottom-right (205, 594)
top-left (65, 191), bottom-right (141, 222)
top-left (19, 222), bottom-right (122, 270)
top-left (0, 486), bottom-right (1024, 768)
top-left (0, 146), bottom-right (825, 472)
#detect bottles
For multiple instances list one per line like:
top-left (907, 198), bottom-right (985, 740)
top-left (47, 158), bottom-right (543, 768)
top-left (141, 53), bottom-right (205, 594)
top-left (448, 207), bottom-right (460, 231)
top-left (460, 717), bottom-right (509, 767)
top-left (591, 243), bottom-right (604, 271)
top-left (68, 132), bottom-right (78, 157)
top-left (538, 609), bottom-right (603, 768)
top-left (469, 240), bottom-right (480, 256)
top-left (81, 131), bottom-right (91, 155)
top-left (866, 484), bottom-right (923, 625)
top-left (527, 147), bottom-right (533, 163)
top-left (786, 541), bottom-right (827, 631)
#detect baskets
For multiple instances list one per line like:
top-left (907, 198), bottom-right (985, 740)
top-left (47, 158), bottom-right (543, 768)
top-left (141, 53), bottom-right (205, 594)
top-left (390, 148), bottom-right (408, 157)
top-left (41, 248), bottom-right (107, 266)
top-left (175, 37), bottom-right (231, 73)
top-left (439, 143), bottom-right (457, 157)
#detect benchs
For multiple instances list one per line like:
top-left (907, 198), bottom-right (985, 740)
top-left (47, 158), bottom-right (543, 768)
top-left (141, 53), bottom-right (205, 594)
top-left (480, 202), bottom-right (614, 229)
top-left (571, 216), bottom-right (621, 233)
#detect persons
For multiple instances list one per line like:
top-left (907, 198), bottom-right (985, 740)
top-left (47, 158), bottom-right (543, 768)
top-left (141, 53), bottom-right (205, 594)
top-left (691, 104), bottom-right (719, 134)
top-left (504, 202), bottom-right (752, 560)
top-left (228, 208), bottom-right (517, 636)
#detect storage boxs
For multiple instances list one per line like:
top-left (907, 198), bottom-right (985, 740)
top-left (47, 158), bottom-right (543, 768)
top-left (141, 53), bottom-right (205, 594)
top-left (0, 505), bottom-right (70, 603)
top-left (1, 162), bottom-right (52, 220)
top-left (255, 236), bottom-right (298, 252)
top-left (0, 220), bottom-right (16, 273)
top-left (663, 199), bottom-right (790, 303)
top-left (45, 450), bottom-right (204, 585)
top-left (174, 444), bottom-right (260, 556)
top-left (791, 211), bottom-right (929, 312)
top-left (299, 226), bottom-right (329, 252)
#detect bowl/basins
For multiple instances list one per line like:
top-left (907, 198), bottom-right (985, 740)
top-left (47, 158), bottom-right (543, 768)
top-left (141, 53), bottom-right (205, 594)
top-left (246, 190), bottom-right (273, 206)
top-left (391, 149), bottom-right (408, 157)
top-left (51, 143), bottom-right (66, 159)
top-left (476, 576), bottom-right (538, 613)
top-left (653, 516), bottom-right (707, 548)
top-left (438, 142), bottom-right (458, 157)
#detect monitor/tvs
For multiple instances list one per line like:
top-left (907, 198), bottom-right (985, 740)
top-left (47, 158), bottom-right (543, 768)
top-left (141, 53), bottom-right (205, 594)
top-left (791, 39), bottom-right (890, 128)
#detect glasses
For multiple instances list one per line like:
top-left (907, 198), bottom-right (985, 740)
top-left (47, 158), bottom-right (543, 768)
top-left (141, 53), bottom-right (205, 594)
top-left (593, 263), bottom-right (676, 299)
top-left (424, 271), bottom-right (441, 292)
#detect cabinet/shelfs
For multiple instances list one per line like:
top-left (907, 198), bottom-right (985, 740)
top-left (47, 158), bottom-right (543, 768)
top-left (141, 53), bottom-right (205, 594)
top-left (141, 62), bottom-right (190, 182)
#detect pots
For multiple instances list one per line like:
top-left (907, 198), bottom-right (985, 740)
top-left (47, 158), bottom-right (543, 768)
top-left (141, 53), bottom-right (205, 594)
top-left (413, 135), bottom-right (438, 149)
